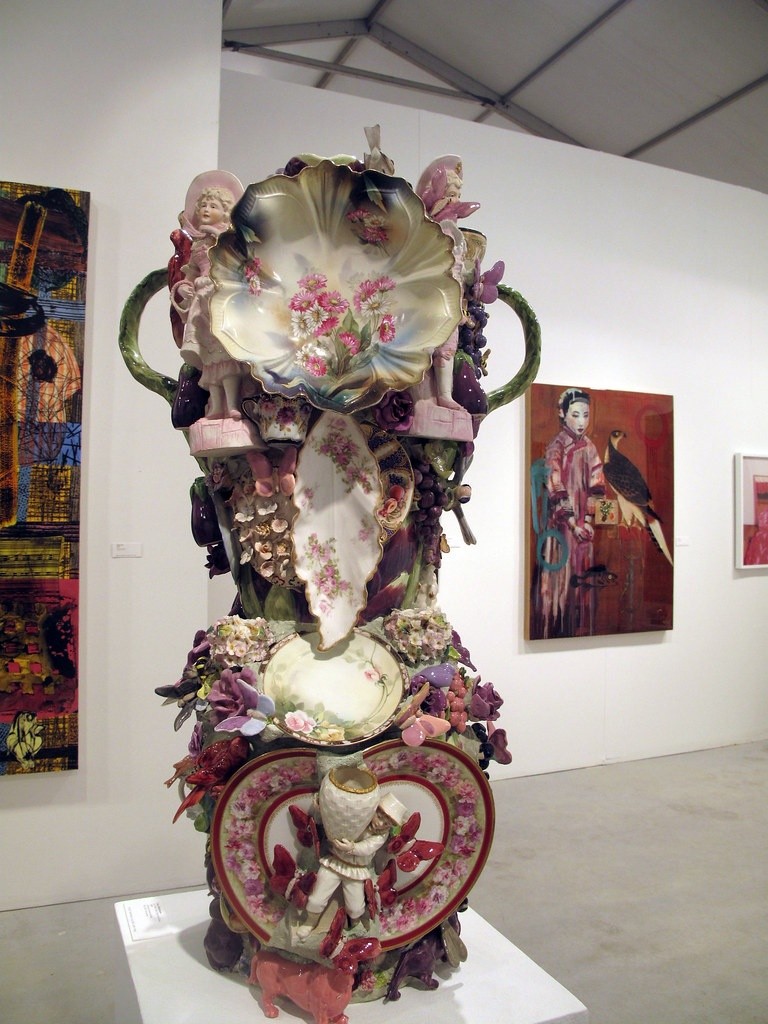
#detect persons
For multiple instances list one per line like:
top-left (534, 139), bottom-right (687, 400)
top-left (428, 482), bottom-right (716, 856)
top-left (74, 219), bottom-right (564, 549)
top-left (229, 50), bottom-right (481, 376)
top-left (415, 154), bottom-right (468, 413)
top-left (296, 792), bottom-right (407, 940)
top-left (174, 170), bottom-right (246, 421)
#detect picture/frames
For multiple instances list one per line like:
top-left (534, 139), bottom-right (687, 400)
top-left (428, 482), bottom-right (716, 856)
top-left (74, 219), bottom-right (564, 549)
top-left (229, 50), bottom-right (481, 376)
top-left (734, 454), bottom-right (768, 569)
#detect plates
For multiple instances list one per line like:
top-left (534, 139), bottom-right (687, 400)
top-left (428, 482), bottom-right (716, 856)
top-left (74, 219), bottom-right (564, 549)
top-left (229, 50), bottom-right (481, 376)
top-left (263, 631), bottom-right (411, 747)
top-left (209, 738), bottom-right (496, 964)
top-left (290, 413), bottom-right (384, 653)
top-left (209, 158), bottom-right (462, 413)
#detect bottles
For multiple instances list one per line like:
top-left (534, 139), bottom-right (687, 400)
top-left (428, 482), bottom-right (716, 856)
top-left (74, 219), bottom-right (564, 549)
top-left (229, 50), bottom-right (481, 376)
top-left (322, 768), bottom-right (381, 844)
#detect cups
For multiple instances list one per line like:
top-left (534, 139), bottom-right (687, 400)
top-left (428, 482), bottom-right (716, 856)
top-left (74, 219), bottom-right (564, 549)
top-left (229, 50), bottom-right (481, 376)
top-left (241, 394), bottom-right (311, 448)
